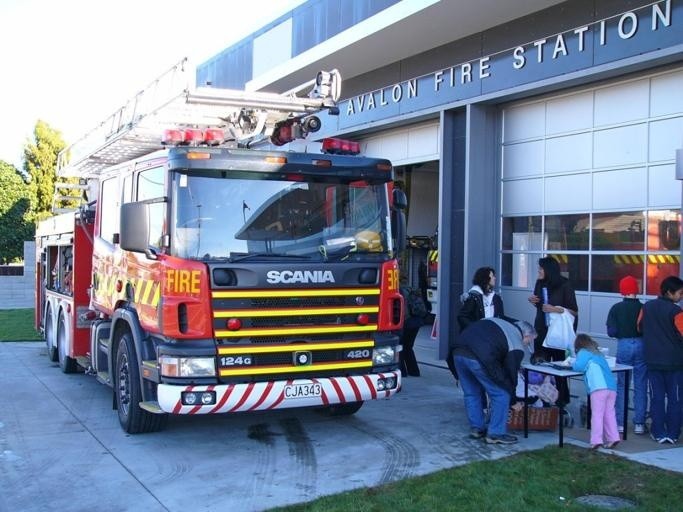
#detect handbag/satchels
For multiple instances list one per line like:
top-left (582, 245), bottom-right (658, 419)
top-left (542, 305), bottom-right (578, 351)
top-left (504, 404), bottom-right (559, 433)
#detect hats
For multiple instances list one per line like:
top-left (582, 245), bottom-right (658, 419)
top-left (619, 275), bottom-right (640, 295)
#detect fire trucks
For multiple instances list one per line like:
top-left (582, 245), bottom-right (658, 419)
top-left (31, 53), bottom-right (407, 434)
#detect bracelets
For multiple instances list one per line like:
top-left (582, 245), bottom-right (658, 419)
top-left (560, 308), bottom-right (564, 313)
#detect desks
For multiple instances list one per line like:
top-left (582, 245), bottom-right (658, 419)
top-left (520, 360), bottom-right (634, 448)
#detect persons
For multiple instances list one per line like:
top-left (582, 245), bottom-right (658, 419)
top-left (449, 315), bottom-right (538, 446)
top-left (525, 255), bottom-right (579, 405)
top-left (570, 333), bottom-right (621, 451)
top-left (633, 275), bottom-right (682, 447)
top-left (397, 275), bottom-right (424, 378)
top-left (455, 266), bottom-right (503, 332)
top-left (604, 274), bottom-right (649, 437)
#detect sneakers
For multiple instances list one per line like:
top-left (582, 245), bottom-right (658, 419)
top-left (469, 426), bottom-right (518, 444)
top-left (591, 423), bottom-right (680, 449)
